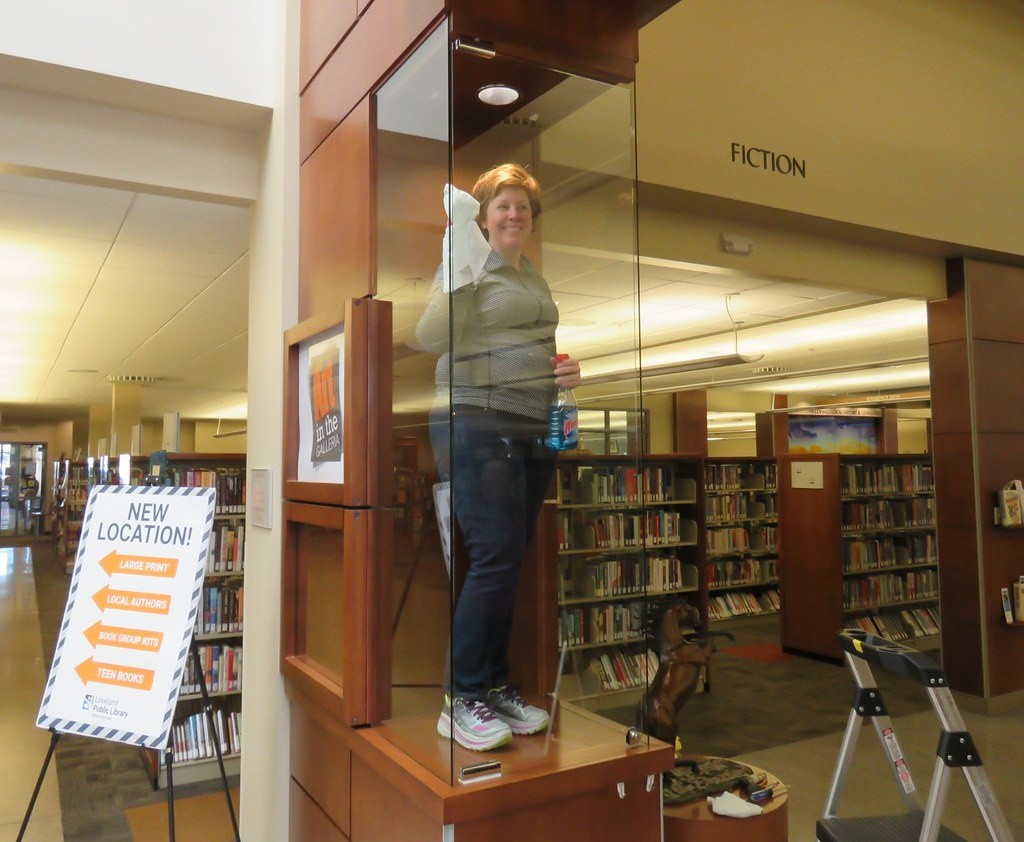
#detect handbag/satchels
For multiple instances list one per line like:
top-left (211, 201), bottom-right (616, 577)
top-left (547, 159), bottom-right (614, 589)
top-left (662, 759), bottom-right (754, 805)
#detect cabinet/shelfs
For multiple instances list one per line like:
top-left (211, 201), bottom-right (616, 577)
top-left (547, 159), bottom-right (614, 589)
top-left (505, 452), bottom-right (711, 713)
top-left (775, 451), bottom-right (939, 667)
top-left (52, 450), bottom-right (248, 789)
top-left (702, 455), bottom-right (783, 632)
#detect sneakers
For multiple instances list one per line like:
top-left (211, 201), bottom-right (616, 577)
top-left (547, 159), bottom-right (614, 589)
top-left (436, 686), bottom-right (551, 753)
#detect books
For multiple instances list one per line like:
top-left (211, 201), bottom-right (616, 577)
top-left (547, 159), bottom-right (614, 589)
top-left (565, 453), bottom-right (785, 700)
top-left (58, 457), bottom-right (245, 769)
top-left (834, 457), bottom-right (941, 645)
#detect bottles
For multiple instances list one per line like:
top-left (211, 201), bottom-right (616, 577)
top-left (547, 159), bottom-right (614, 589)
top-left (548, 354), bottom-right (579, 449)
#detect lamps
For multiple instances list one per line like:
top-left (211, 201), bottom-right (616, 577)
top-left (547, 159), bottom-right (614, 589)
top-left (573, 293), bottom-right (763, 386)
top-left (211, 417), bottom-right (249, 439)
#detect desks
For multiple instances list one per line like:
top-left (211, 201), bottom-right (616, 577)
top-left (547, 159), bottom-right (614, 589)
top-left (32, 512), bottom-right (51, 543)
top-left (660, 755), bottom-right (790, 842)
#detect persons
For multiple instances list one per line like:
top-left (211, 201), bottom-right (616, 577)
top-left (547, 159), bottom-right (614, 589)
top-left (418, 165), bottom-right (561, 753)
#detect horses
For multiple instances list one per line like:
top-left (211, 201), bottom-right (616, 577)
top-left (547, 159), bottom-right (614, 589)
top-left (632, 592), bottom-right (717, 784)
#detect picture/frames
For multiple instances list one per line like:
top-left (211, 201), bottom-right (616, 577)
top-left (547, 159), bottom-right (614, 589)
top-left (279, 297), bottom-right (369, 506)
top-left (279, 500), bottom-right (368, 725)
top-left (375, 508), bottom-right (395, 721)
top-left (371, 299), bottom-right (394, 506)
